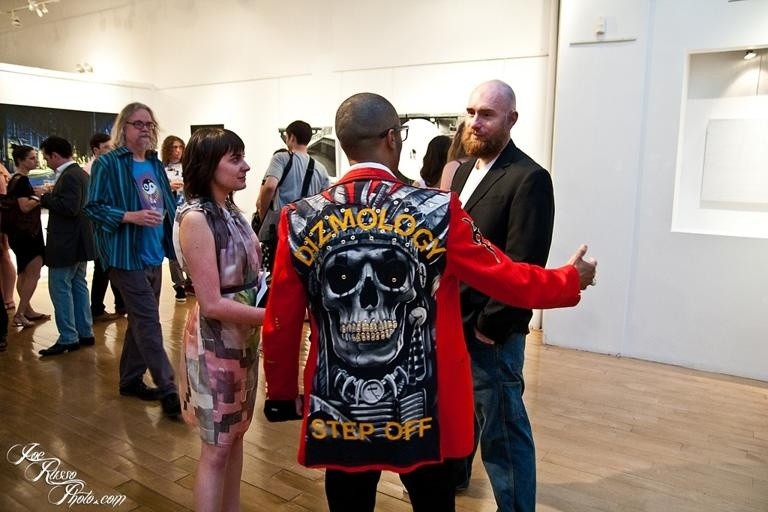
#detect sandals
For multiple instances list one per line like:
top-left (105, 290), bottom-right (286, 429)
top-left (11, 312), bottom-right (35, 329)
top-left (3, 302), bottom-right (14, 310)
top-left (24, 314), bottom-right (51, 322)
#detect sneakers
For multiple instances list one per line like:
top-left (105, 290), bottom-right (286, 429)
top-left (185, 285), bottom-right (194, 295)
top-left (173, 283), bottom-right (187, 302)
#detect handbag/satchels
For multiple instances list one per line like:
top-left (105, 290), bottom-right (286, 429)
top-left (251, 148), bottom-right (294, 233)
top-left (260, 154), bottom-right (315, 245)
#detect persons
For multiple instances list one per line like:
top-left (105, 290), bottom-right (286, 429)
top-left (7, 145), bottom-right (50, 328)
top-left (261, 93), bottom-right (597, 512)
top-left (413, 134), bottom-right (452, 188)
top-left (260, 121), bottom-right (329, 275)
top-left (83, 133), bottom-right (125, 324)
top-left (160, 135), bottom-right (195, 303)
top-left (85, 102), bottom-right (180, 420)
top-left (33, 135), bottom-right (95, 357)
top-left (0, 162), bottom-right (16, 353)
top-left (440, 120), bottom-right (470, 192)
top-left (176, 128), bottom-right (309, 512)
top-left (444, 80), bottom-right (554, 512)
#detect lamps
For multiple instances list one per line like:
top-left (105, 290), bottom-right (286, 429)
top-left (34, 5), bottom-right (43, 18)
top-left (11, 11), bottom-right (22, 30)
top-left (27, 1), bottom-right (33, 11)
top-left (42, 2), bottom-right (48, 14)
top-left (745, 49), bottom-right (757, 60)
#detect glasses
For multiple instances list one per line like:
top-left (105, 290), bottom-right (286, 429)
top-left (126, 120), bottom-right (156, 130)
top-left (378, 126), bottom-right (409, 142)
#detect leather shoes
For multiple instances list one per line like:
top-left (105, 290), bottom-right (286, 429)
top-left (120, 382), bottom-right (159, 401)
top-left (79, 336), bottom-right (94, 346)
top-left (93, 310), bottom-right (120, 323)
top-left (39, 341), bottom-right (80, 355)
top-left (160, 393), bottom-right (181, 419)
